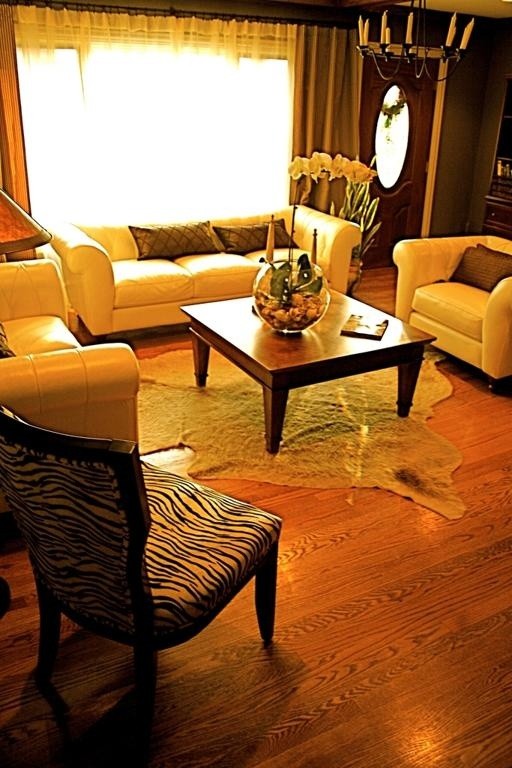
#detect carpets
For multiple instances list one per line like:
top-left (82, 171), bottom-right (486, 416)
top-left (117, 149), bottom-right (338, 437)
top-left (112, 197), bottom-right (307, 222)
top-left (131, 345), bottom-right (467, 522)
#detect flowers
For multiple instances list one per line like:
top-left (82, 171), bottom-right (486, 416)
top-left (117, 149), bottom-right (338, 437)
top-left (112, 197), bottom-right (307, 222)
top-left (255, 148), bottom-right (378, 303)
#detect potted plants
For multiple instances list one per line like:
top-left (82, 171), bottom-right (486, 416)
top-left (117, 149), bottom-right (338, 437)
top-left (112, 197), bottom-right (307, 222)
top-left (329, 154), bottom-right (384, 297)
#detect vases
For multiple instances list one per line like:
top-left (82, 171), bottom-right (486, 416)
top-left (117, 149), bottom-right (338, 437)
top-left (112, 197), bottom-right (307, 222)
top-left (251, 261), bottom-right (331, 339)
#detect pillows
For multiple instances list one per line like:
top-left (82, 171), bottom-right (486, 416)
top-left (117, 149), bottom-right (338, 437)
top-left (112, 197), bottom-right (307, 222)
top-left (450, 246), bottom-right (512, 292)
top-left (131, 221), bottom-right (221, 261)
top-left (213, 218), bottom-right (300, 254)
top-left (0, 323), bottom-right (15, 357)
top-left (475, 239), bottom-right (510, 259)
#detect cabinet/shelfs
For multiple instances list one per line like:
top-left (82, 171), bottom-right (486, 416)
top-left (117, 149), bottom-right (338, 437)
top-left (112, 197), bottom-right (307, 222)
top-left (479, 72), bottom-right (512, 239)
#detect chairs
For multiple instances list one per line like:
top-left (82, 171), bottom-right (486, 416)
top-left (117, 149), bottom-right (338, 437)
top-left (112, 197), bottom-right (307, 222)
top-left (0, 256), bottom-right (147, 515)
top-left (390, 232), bottom-right (512, 394)
top-left (0, 400), bottom-right (283, 738)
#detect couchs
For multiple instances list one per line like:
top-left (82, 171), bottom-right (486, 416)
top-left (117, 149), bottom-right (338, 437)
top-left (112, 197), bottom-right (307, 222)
top-left (46, 204), bottom-right (365, 349)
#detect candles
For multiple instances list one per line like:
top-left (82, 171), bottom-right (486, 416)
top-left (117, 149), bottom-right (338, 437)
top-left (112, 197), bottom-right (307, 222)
top-left (356, 9), bottom-right (477, 53)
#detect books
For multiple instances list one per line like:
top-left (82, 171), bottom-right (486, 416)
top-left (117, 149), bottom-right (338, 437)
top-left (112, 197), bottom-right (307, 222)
top-left (340, 313), bottom-right (389, 341)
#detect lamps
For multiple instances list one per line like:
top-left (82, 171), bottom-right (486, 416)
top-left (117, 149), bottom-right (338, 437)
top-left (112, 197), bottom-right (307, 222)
top-left (0, 186), bottom-right (52, 260)
